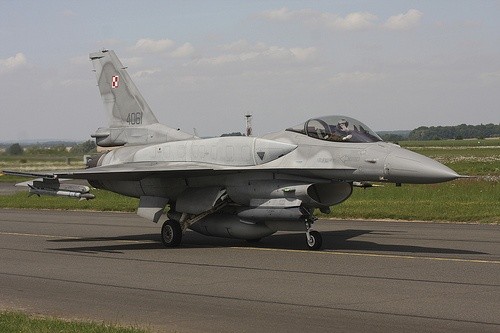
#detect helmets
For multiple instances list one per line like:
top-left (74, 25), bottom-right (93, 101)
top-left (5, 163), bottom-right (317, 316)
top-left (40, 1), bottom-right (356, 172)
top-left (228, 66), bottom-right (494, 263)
top-left (337, 119), bottom-right (349, 129)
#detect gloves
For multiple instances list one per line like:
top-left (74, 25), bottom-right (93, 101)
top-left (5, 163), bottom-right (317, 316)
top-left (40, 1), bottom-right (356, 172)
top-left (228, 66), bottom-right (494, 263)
top-left (343, 134), bottom-right (353, 141)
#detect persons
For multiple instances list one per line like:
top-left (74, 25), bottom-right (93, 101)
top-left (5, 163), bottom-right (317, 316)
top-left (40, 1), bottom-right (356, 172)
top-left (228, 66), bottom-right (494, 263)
top-left (337, 119), bottom-right (363, 143)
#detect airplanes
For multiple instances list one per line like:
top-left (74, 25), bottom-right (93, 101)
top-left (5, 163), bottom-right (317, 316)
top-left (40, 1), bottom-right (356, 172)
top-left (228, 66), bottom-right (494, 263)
top-left (2, 48), bottom-right (478, 251)
